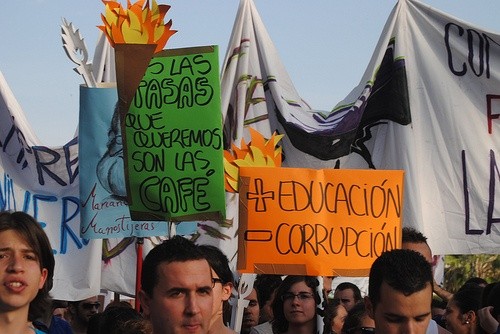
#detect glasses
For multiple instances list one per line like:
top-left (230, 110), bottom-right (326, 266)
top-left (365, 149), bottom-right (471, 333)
top-left (211, 277), bottom-right (222, 288)
top-left (352, 326), bottom-right (375, 334)
top-left (83, 302), bottom-right (100, 310)
top-left (280, 292), bottom-right (314, 302)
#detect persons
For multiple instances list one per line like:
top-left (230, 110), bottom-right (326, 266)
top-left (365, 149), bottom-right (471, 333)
top-left (0, 209), bottom-right (500, 334)
